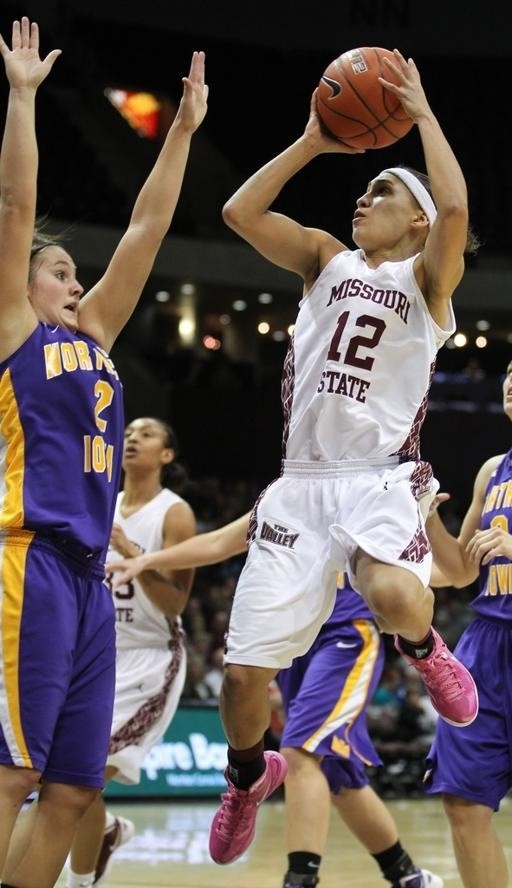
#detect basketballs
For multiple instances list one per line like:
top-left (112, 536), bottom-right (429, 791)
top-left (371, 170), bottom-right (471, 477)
top-left (317, 47), bottom-right (414, 149)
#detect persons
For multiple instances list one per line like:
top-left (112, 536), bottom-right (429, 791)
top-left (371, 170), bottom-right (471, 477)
top-left (207, 45), bottom-right (481, 867)
top-left (0, 16), bottom-right (214, 888)
top-left (181, 474), bottom-right (477, 799)
top-left (0, 414), bottom-right (197, 888)
top-left (423, 356), bottom-right (512, 888)
top-left (103, 510), bottom-right (445, 887)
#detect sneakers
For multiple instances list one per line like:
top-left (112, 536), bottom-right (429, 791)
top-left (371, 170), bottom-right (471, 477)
top-left (391, 866), bottom-right (444, 887)
top-left (395, 623), bottom-right (479, 728)
top-left (208, 750), bottom-right (288, 867)
top-left (93, 813), bottom-right (136, 883)
top-left (282, 869), bottom-right (320, 888)
